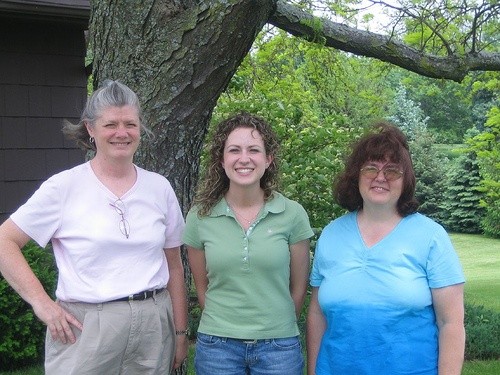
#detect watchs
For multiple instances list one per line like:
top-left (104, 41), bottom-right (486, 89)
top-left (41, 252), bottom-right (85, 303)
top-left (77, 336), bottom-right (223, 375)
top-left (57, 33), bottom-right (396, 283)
top-left (176, 327), bottom-right (191, 336)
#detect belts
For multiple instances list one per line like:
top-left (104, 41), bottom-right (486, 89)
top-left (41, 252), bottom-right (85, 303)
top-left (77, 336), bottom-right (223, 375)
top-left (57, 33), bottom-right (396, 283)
top-left (107, 288), bottom-right (164, 302)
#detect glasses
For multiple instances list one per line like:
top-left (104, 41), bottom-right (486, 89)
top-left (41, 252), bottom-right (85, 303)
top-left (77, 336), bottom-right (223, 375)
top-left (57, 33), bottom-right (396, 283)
top-left (360, 166), bottom-right (404, 181)
top-left (109, 198), bottom-right (131, 238)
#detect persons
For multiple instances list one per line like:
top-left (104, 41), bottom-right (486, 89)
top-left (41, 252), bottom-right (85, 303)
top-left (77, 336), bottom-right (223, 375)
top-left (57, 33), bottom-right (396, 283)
top-left (1, 79), bottom-right (186, 375)
top-left (183, 114), bottom-right (314, 375)
top-left (307, 123), bottom-right (468, 375)
